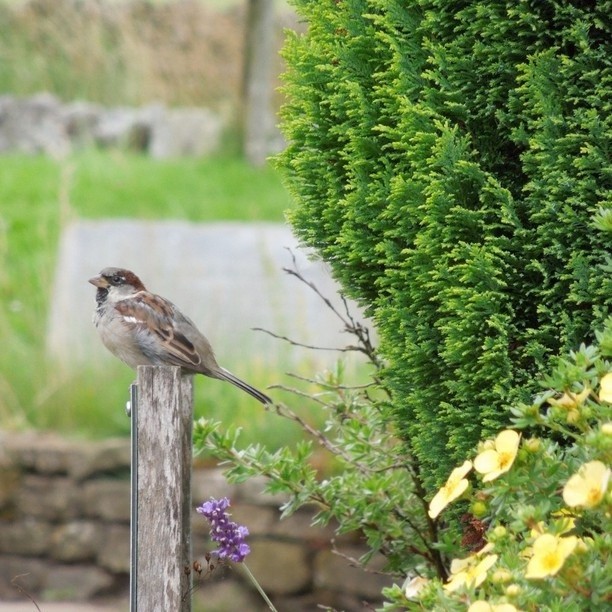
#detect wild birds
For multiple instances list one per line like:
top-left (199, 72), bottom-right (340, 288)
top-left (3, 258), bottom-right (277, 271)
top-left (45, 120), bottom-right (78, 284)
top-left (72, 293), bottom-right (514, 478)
top-left (86, 267), bottom-right (272, 408)
